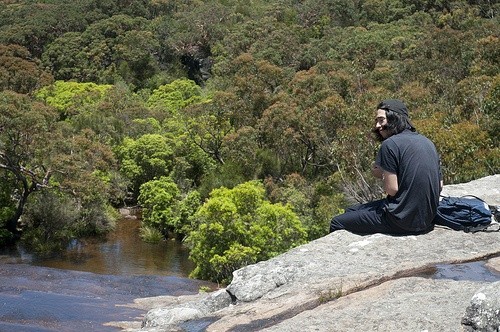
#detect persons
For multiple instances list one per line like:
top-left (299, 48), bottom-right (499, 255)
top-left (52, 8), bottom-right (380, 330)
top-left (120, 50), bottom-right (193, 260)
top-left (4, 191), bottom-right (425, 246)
top-left (329, 99), bottom-right (440, 235)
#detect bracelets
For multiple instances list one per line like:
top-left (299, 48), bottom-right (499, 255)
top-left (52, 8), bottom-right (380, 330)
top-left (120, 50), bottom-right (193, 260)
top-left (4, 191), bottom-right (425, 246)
top-left (380, 169), bottom-right (385, 182)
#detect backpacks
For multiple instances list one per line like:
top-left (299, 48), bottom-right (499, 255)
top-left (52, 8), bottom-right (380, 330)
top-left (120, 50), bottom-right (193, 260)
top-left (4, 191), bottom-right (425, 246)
top-left (436, 194), bottom-right (492, 232)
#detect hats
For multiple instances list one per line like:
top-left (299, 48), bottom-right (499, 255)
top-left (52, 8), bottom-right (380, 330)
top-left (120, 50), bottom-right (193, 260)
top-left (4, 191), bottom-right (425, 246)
top-left (377, 100), bottom-right (409, 116)
